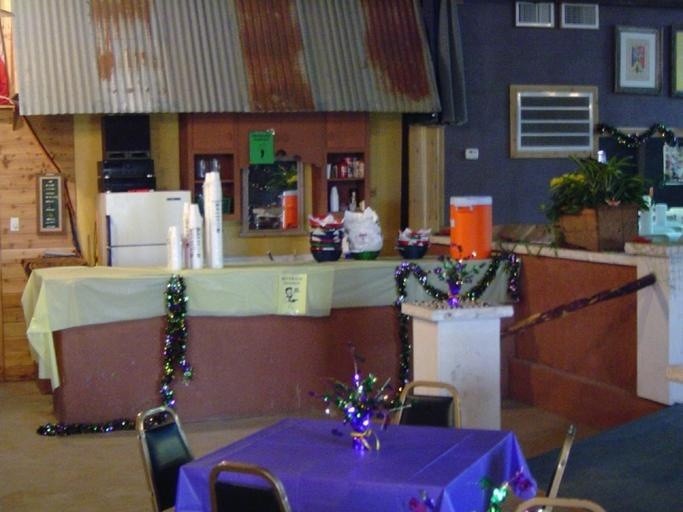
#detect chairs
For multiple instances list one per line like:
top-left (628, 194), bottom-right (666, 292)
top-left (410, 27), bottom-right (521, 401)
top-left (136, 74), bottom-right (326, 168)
top-left (515, 497), bottom-right (606, 512)
top-left (137, 405), bottom-right (195, 512)
top-left (535, 423), bottom-right (577, 512)
top-left (206, 464), bottom-right (292, 512)
top-left (393, 378), bottom-right (462, 430)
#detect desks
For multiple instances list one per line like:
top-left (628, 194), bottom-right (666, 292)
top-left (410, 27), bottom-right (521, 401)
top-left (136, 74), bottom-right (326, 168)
top-left (174, 421), bottom-right (535, 512)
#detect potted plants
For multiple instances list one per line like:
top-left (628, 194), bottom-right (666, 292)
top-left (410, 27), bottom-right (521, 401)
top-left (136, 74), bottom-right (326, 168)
top-left (537, 153), bottom-right (649, 251)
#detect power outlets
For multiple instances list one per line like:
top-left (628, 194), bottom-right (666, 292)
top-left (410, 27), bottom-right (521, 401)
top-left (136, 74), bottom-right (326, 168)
top-left (9, 216), bottom-right (20, 231)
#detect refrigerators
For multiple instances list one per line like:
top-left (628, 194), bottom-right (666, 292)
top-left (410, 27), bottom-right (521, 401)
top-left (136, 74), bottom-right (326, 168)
top-left (99, 189), bottom-right (191, 267)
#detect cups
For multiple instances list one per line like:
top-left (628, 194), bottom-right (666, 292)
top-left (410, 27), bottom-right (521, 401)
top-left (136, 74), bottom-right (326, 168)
top-left (165, 166), bottom-right (224, 272)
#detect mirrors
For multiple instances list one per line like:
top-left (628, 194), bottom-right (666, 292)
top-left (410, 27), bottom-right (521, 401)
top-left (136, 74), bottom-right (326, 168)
top-left (239, 154), bottom-right (305, 235)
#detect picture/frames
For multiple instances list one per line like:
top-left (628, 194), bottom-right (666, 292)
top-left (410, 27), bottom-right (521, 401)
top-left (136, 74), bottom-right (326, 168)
top-left (613, 24), bottom-right (662, 95)
top-left (37, 175), bottom-right (63, 233)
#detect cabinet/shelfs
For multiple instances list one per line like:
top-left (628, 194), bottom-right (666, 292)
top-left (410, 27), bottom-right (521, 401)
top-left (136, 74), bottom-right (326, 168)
top-left (188, 149), bottom-right (240, 220)
top-left (323, 145), bottom-right (369, 216)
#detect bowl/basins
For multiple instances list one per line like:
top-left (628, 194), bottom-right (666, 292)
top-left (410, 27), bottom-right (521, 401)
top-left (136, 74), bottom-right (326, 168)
top-left (307, 214), bottom-right (344, 263)
top-left (352, 251), bottom-right (380, 261)
top-left (392, 228), bottom-right (429, 261)
